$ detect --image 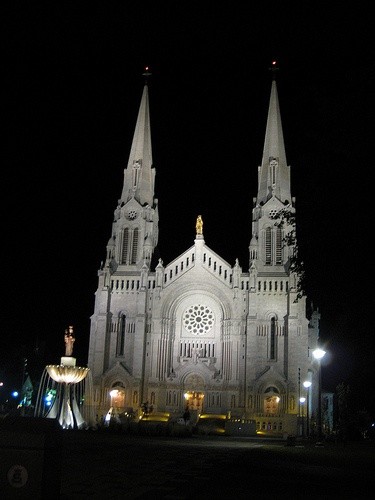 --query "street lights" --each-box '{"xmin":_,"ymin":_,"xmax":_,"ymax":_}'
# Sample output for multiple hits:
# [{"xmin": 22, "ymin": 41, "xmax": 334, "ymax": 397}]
[
  {"xmin": 313, "ymin": 346, "xmax": 326, "ymax": 448},
  {"xmin": 303, "ymin": 380, "xmax": 311, "ymax": 444},
  {"xmin": 299, "ymin": 396, "xmax": 306, "ymax": 442}
]
